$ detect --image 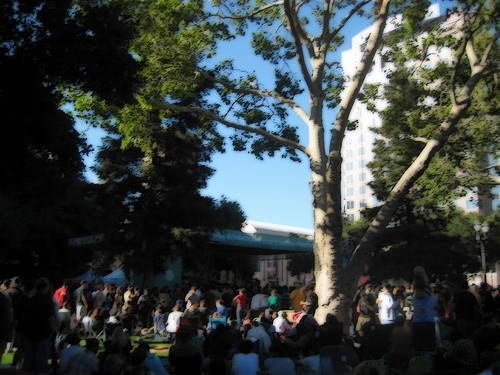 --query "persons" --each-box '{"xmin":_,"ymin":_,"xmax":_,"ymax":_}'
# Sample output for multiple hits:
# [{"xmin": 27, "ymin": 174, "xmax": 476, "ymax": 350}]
[{"xmin": 1, "ymin": 264, "xmax": 499, "ymax": 375}]
